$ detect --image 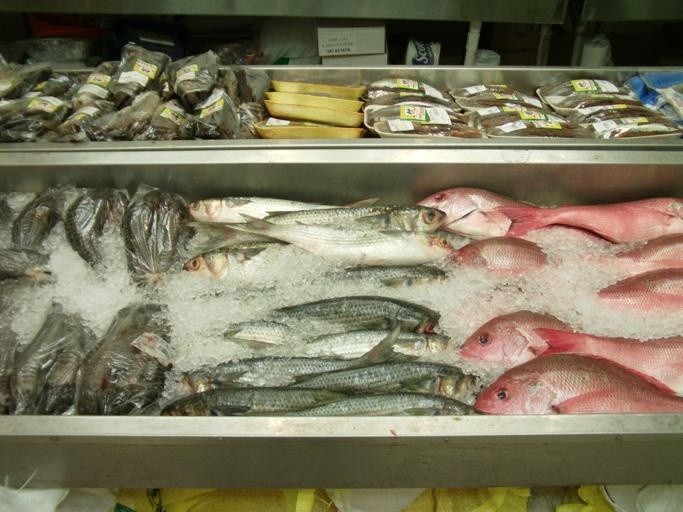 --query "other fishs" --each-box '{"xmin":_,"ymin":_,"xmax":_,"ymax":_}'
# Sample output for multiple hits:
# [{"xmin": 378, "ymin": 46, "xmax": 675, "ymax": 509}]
[{"xmin": 0, "ymin": 183, "xmax": 683, "ymax": 419}]
